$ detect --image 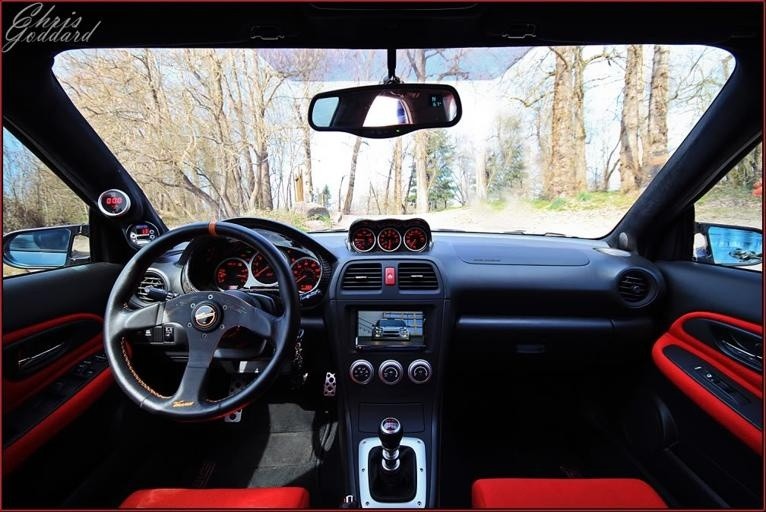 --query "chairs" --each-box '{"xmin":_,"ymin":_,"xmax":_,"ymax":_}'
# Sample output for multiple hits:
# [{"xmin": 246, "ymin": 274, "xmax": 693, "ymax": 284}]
[
  {"xmin": 470, "ymin": 478, "xmax": 669, "ymax": 509},
  {"xmin": 120, "ymin": 487, "xmax": 309, "ymax": 508}
]
